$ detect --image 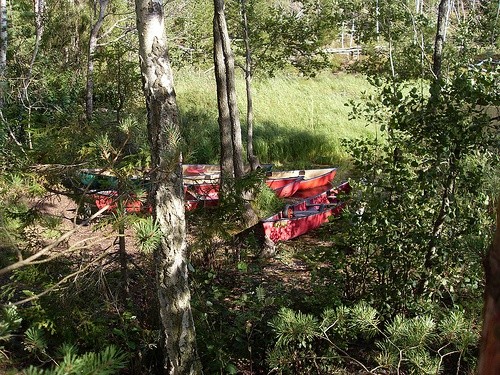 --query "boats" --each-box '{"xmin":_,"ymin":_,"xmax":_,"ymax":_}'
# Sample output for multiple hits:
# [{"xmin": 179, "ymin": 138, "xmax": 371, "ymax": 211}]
[{"xmin": 53, "ymin": 155, "xmax": 354, "ymax": 247}]
